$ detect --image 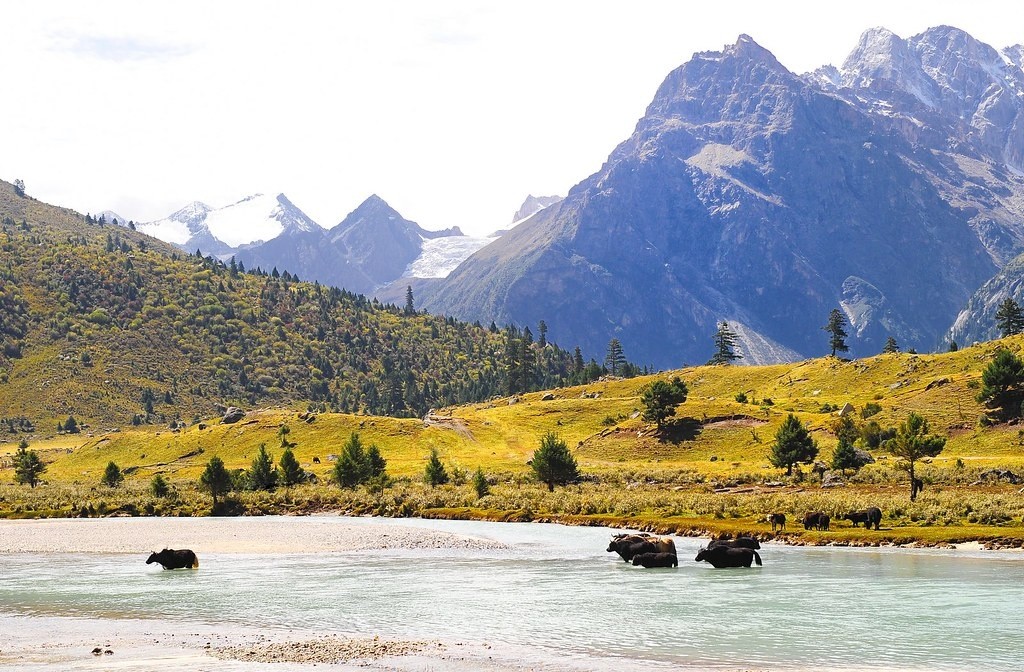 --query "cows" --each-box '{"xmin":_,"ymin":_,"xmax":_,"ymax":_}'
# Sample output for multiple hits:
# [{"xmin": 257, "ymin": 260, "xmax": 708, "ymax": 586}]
[
  {"xmin": 766, "ymin": 506, "xmax": 883, "ymax": 532},
  {"xmin": 606, "ymin": 532, "xmax": 762, "ymax": 569},
  {"xmin": 312, "ymin": 457, "xmax": 320, "ymax": 463},
  {"xmin": 146, "ymin": 549, "xmax": 199, "ymax": 569}
]
